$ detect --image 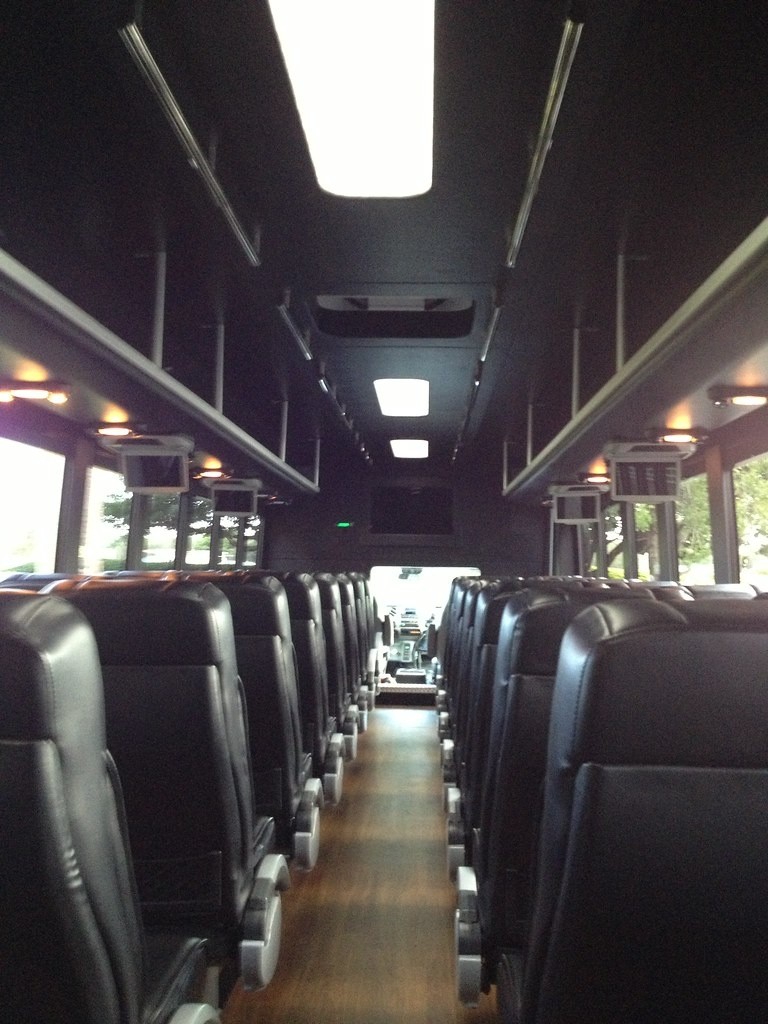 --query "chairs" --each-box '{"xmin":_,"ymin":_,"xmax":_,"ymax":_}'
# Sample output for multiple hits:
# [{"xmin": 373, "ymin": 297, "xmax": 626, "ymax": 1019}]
[{"xmin": 0, "ymin": 563, "xmax": 768, "ymax": 1020}]
